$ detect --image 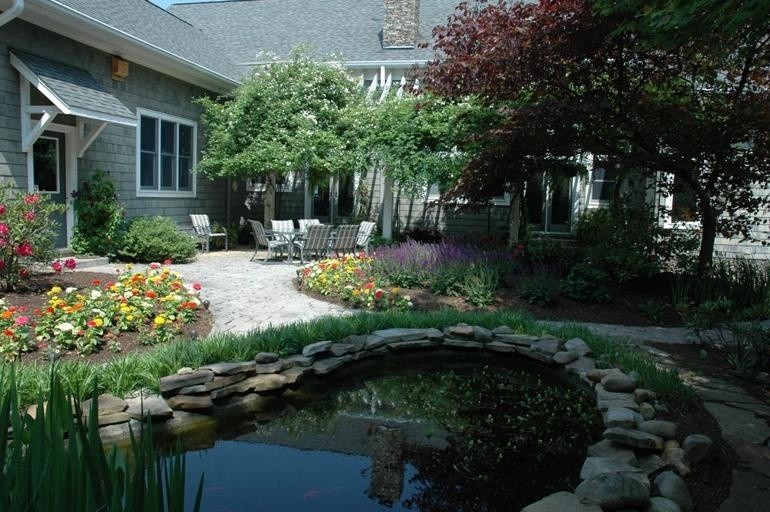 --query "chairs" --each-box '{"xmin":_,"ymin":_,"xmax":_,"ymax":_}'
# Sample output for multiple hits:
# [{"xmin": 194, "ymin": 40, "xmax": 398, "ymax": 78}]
[
  {"xmin": 247, "ymin": 219, "xmax": 378, "ymax": 265},
  {"xmin": 189, "ymin": 213, "xmax": 228, "ymax": 252}
]
